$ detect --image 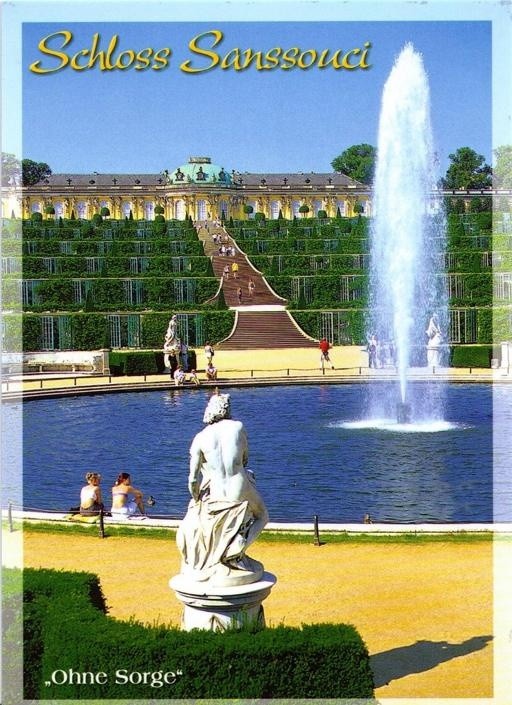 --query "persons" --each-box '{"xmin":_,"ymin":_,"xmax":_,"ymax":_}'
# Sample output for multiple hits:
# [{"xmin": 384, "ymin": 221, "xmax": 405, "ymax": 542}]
[
  {"xmin": 190, "ymin": 369, "xmax": 200, "ymax": 387},
  {"xmin": 193, "ymin": 216, "xmax": 256, "ymax": 302},
  {"xmin": 147, "ymin": 496, "xmax": 155, "ymax": 506},
  {"xmin": 80, "ymin": 472, "xmax": 104, "ymax": 516},
  {"xmin": 205, "ymin": 363, "xmax": 217, "ymax": 382},
  {"xmin": 205, "ymin": 341, "xmax": 213, "ymax": 363},
  {"xmin": 320, "ymin": 337, "xmax": 334, "ymax": 374},
  {"xmin": 188, "ymin": 393, "xmax": 268, "ymax": 573},
  {"xmin": 169, "ymin": 343, "xmax": 187, "ymax": 386},
  {"xmin": 368, "ymin": 335, "xmax": 386, "ymax": 369},
  {"xmin": 111, "ymin": 472, "xmax": 145, "ymax": 518},
  {"xmin": 166, "ymin": 315, "xmax": 180, "ymax": 346}
]
